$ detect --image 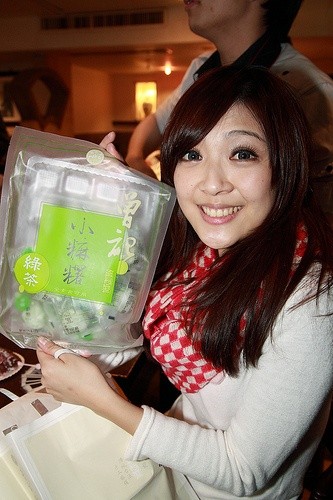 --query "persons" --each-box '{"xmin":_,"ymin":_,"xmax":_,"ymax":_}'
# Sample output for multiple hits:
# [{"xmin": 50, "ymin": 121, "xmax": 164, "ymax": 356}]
[
  {"xmin": 97, "ymin": 0, "xmax": 332, "ymax": 263},
  {"xmin": 37, "ymin": 65, "xmax": 333, "ymax": 500}
]
